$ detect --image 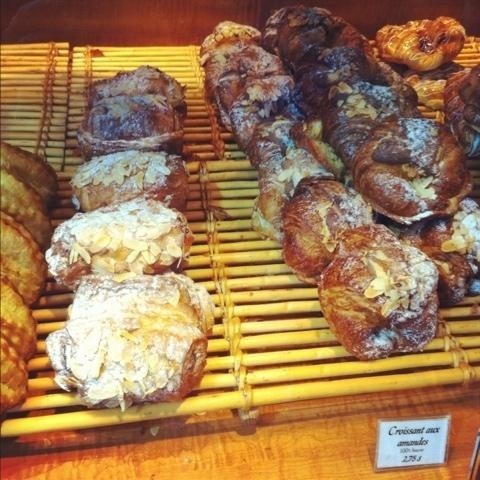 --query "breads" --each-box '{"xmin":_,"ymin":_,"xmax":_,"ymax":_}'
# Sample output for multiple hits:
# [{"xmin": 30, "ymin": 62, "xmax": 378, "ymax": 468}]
[
  {"xmin": 45, "ymin": 272, "xmax": 215, "ymax": 410},
  {"xmin": 216, "ymin": 45, "xmax": 290, "ymax": 133},
  {"xmin": 278, "ymin": 176, "xmax": 375, "ymax": 286},
  {"xmin": 228, "ymin": 82, "xmax": 303, "ymax": 152},
  {"xmin": 352, "ymin": 117, "xmax": 474, "ymax": 226},
  {"xmin": 0, "ymin": 282, "xmax": 37, "ymax": 361},
  {"xmin": 1, "ymin": 169, "xmax": 53, "ymax": 253},
  {"xmin": 87, "ymin": 64, "xmax": 187, "ymax": 121},
  {"xmin": 0, "ymin": 338, "xmax": 30, "ymax": 412},
  {"xmin": 249, "ymin": 148, "xmax": 337, "ymax": 245},
  {"xmin": 316, "ymin": 223, "xmax": 440, "ymax": 362},
  {"xmin": 1, "ymin": 210, "xmax": 48, "ymax": 307},
  {"xmin": 442, "ymin": 63, "xmax": 480, "ymax": 159},
  {"xmin": 295, "ymin": 46, "xmax": 393, "ymax": 116},
  {"xmin": 44, "ymin": 198, "xmax": 196, "ymax": 296},
  {"xmin": 291, "ymin": 117, "xmax": 345, "ymax": 176},
  {"xmin": 263, "ymin": 4, "xmax": 333, "ymax": 56},
  {"xmin": 397, "ymin": 196, "xmax": 480, "ymax": 308},
  {"xmin": 278, "ymin": 11, "xmax": 363, "ymax": 74},
  {"xmin": 205, "ymin": 37, "xmax": 262, "ymax": 99},
  {"xmin": 375, "ymin": 15, "xmax": 466, "ymax": 71},
  {"xmin": 67, "ymin": 149, "xmax": 190, "ymax": 212},
  {"xmin": 246, "ymin": 114, "xmax": 309, "ymax": 170},
  {"xmin": 198, "ymin": 21, "xmax": 263, "ymax": 68},
  {"xmin": 76, "ymin": 95, "xmax": 186, "ymax": 163},
  {"xmin": 321, "ymin": 81, "xmax": 419, "ymax": 168},
  {"xmin": 1, "ymin": 143, "xmax": 59, "ymax": 207},
  {"xmin": 403, "ymin": 62, "xmax": 465, "ymax": 125}
]
